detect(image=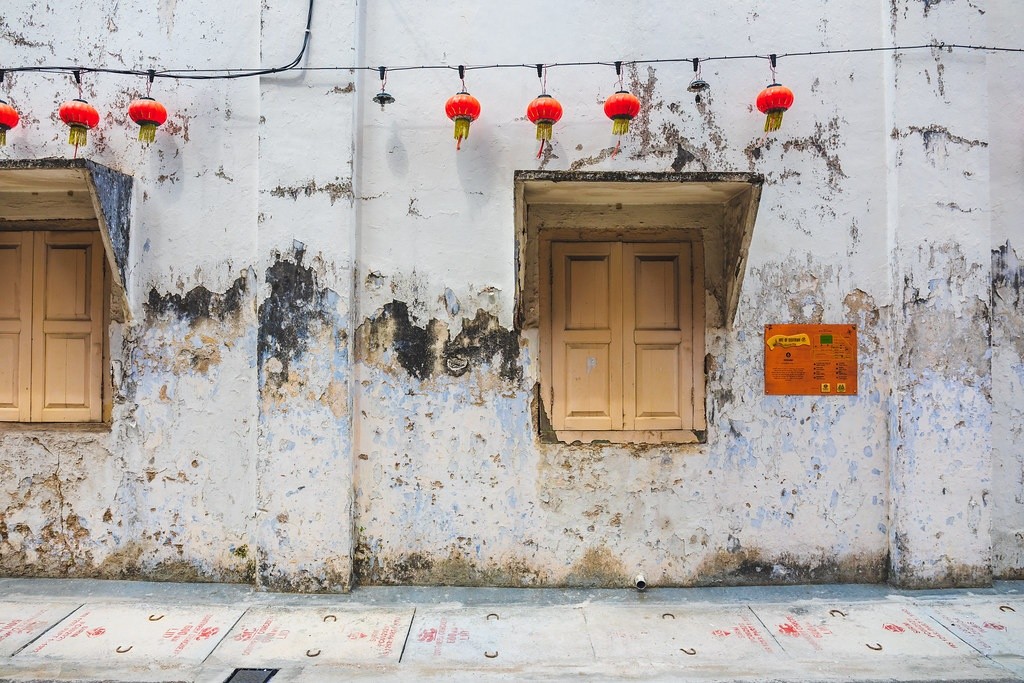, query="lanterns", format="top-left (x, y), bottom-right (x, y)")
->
top-left (128, 96), bottom-right (167, 143)
top-left (59, 99), bottom-right (100, 158)
top-left (444, 92), bottom-right (480, 150)
top-left (527, 94), bottom-right (563, 158)
top-left (0, 100), bottom-right (19, 146)
top-left (757, 81), bottom-right (794, 134)
top-left (604, 90), bottom-right (640, 158)
